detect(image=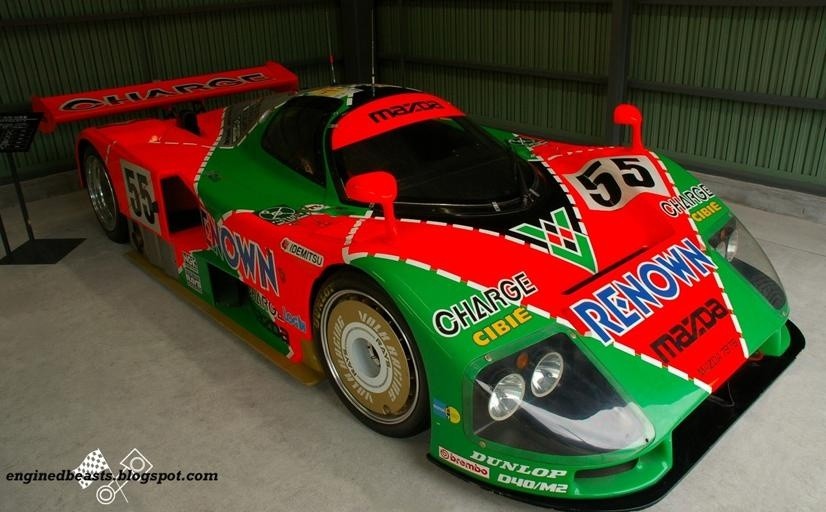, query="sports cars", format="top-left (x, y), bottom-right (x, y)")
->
top-left (30, 60), bottom-right (806, 507)
